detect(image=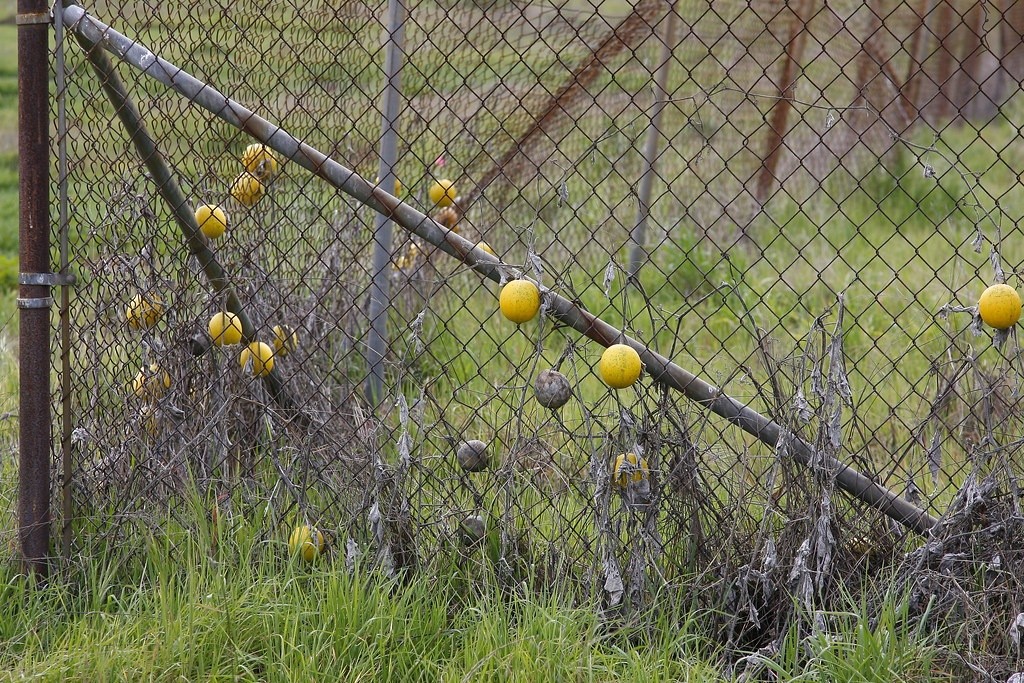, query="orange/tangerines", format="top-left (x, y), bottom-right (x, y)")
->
top-left (125, 141), bottom-right (1021, 560)
